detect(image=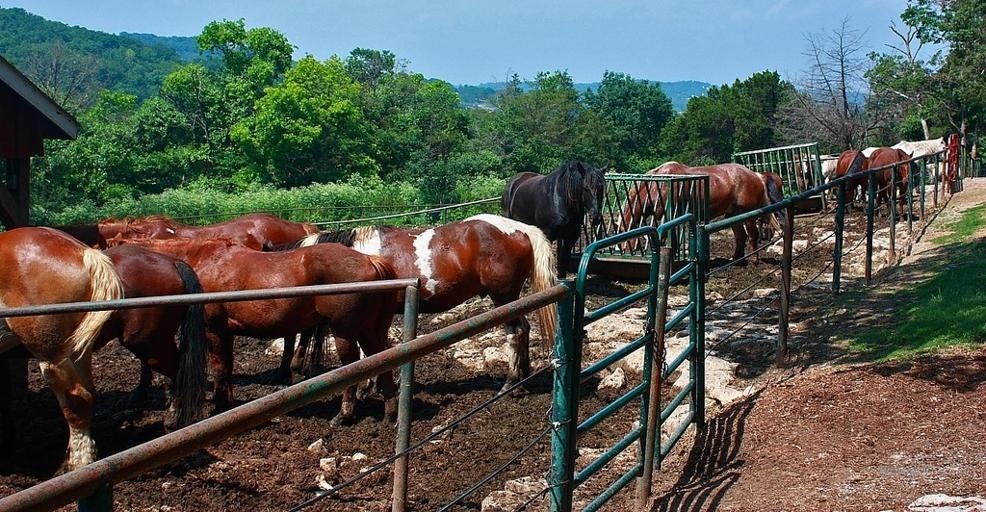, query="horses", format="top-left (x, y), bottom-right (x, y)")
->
top-left (258, 209), bottom-right (560, 392)
top-left (613, 161), bottom-right (779, 267)
top-left (0, 224), bottom-right (129, 473)
top-left (790, 136), bottom-right (950, 222)
top-left (498, 159), bottom-right (611, 280)
top-left (97, 211), bottom-right (324, 372)
top-left (104, 231), bottom-right (397, 427)
top-left (757, 164), bottom-right (787, 222)
top-left (85, 242), bottom-right (207, 427)
